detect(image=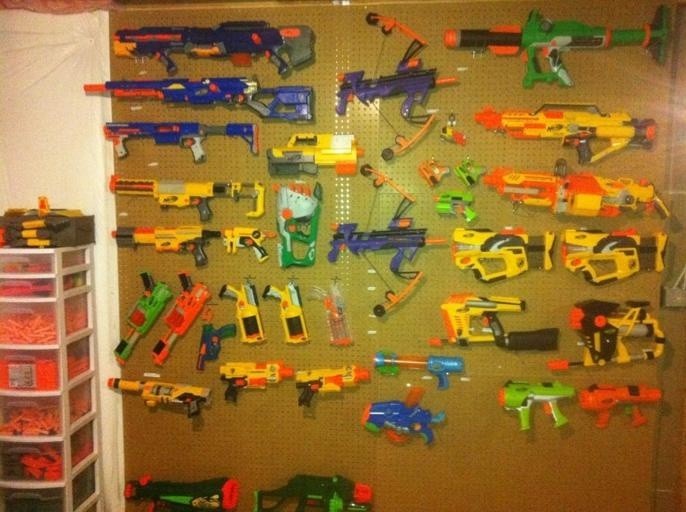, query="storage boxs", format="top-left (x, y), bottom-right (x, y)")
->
top-left (1, 244), bottom-right (107, 512)
